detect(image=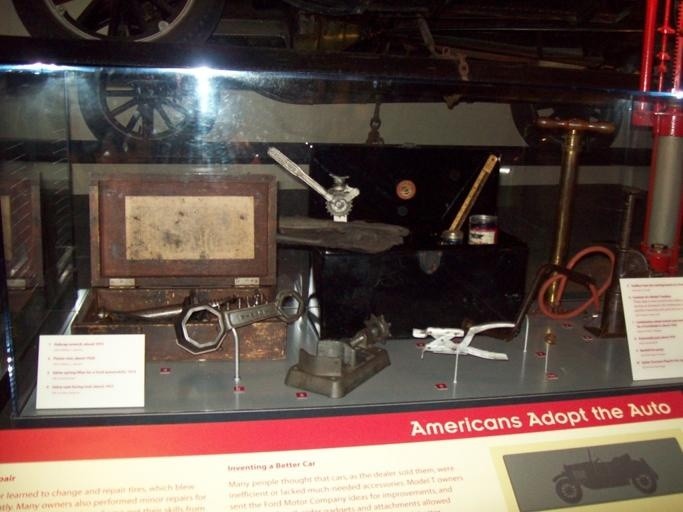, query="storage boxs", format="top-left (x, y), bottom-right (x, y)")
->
top-left (72, 172), bottom-right (287, 362)
top-left (310, 143), bottom-right (529, 341)
